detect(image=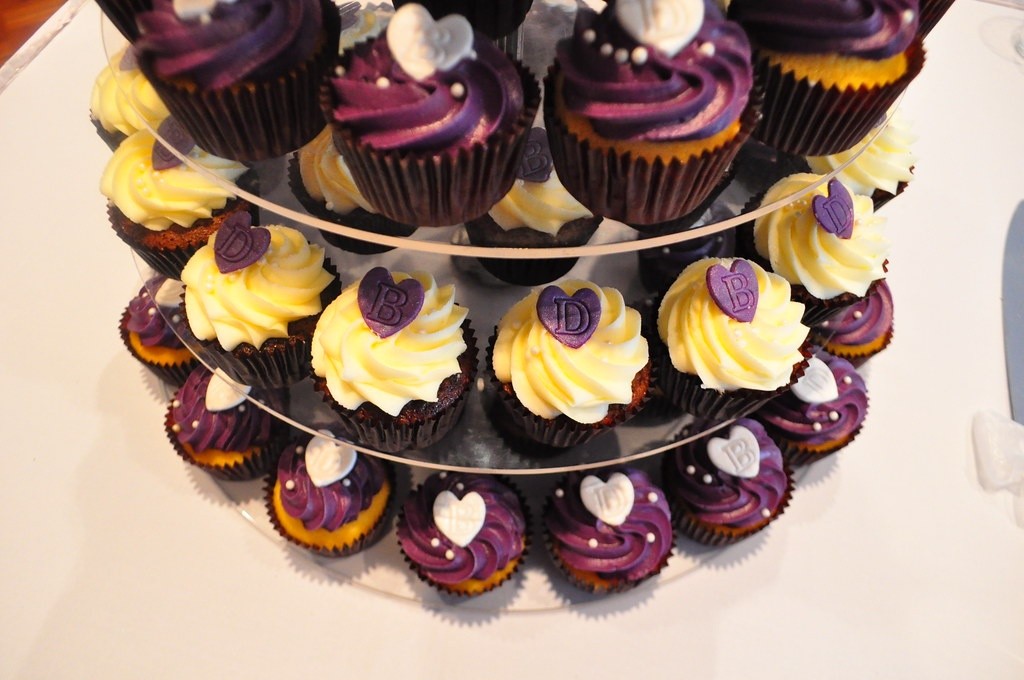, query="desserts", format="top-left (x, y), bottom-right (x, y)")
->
top-left (88, 0), bottom-right (927, 599)
